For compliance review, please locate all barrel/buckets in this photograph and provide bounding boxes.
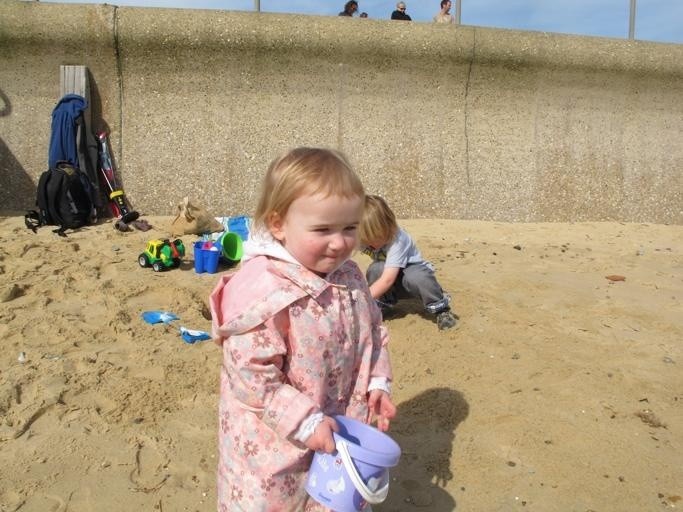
[214,232,243,267]
[301,414,403,512]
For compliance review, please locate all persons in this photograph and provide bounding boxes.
[359,192,459,332]
[338,1,368,19]
[433,0,456,23]
[390,2,411,20]
[208,147,399,512]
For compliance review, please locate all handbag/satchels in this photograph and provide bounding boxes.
[171,196,224,235]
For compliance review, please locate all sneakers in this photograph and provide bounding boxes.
[437,312,455,330]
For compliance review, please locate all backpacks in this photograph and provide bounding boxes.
[26,160,97,237]
